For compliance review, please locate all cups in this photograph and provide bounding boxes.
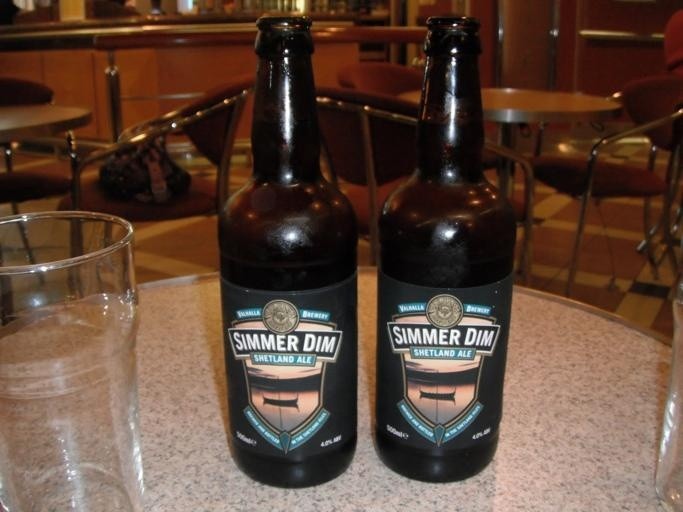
[655,299,683,511]
[1,210,148,511]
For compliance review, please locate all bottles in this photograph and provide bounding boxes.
[374,14,518,482]
[217,10,360,490]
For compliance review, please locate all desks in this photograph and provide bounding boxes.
[0,105,92,293]
[0,268,683,512]
[398,84,624,224]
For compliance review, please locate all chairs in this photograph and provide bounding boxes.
[535,76,682,298]
[339,61,518,196]
[1,79,53,283]
[314,86,545,280]
[55,75,258,292]
